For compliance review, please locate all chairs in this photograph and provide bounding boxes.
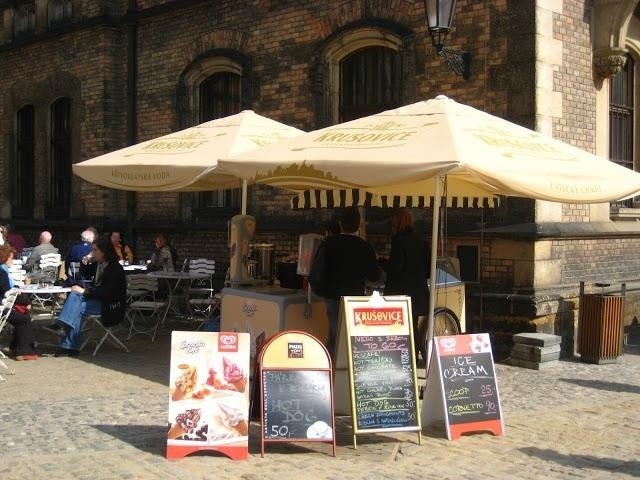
[1,247,230,381]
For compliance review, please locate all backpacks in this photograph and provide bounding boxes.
[308,235,336,298]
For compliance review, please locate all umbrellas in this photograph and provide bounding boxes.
[218,95,640,381]
[70,110,307,216]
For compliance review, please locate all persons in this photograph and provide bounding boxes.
[310,206,376,352]
[141,235,173,317]
[54,233,126,356]
[324,220,341,240]
[0,246,40,360]
[382,206,430,369]
[0,223,134,294]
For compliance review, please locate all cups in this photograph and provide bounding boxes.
[24,275,56,290]
[313,420,329,438]
[119,256,151,267]
[82,252,96,265]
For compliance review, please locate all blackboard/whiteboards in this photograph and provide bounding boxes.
[259,330,336,442]
[344,296,422,433]
[434,333,504,440]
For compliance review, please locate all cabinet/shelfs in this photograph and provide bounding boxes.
[365,254,467,343]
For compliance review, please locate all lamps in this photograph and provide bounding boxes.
[423,0,472,85]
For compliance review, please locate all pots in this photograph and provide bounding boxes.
[249,242,276,286]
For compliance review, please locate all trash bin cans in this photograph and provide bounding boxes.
[576,281,627,365]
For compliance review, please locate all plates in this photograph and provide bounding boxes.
[306,424,332,439]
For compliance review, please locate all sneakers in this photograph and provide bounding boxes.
[47,322,68,333]
[15,353,39,361]
[54,348,78,357]
